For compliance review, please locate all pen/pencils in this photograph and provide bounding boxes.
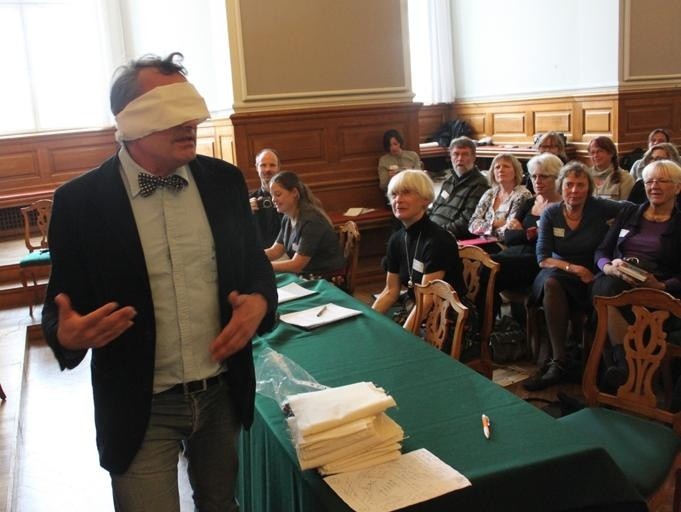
[317,307,326,317]
[481,414,491,440]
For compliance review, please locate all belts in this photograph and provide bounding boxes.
[160,375,221,395]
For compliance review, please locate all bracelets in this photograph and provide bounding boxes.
[493,220,497,228]
[566,262,572,273]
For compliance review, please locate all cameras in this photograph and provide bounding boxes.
[257,196,272,210]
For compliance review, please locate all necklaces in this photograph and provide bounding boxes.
[565,207,583,221]
[404,229,424,290]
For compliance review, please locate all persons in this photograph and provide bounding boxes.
[524,126,681,205]
[370,169,480,362]
[371,135,489,307]
[591,160,681,393]
[376,128,421,206]
[467,151,564,346]
[247,147,284,250]
[460,152,533,328]
[264,169,346,291]
[40,51,281,512]
[522,159,637,392]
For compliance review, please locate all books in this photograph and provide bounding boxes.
[274,282,319,304]
[278,302,364,331]
[617,261,649,282]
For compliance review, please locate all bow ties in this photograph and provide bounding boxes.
[137,172,189,198]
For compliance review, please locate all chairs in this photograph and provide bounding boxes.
[555,287,680,510]
[412,279,469,361]
[661,329,680,411]
[324,225,359,293]
[19,199,54,316]
[458,245,500,380]
[532,305,593,365]
[499,285,532,362]
[235,273,649,511]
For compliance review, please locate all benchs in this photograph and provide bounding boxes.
[327,206,393,235]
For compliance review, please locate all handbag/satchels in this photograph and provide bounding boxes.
[490,328,528,362]
[495,229,535,287]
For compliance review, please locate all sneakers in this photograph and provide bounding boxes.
[542,355,572,380]
[523,358,560,390]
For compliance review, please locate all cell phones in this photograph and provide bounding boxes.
[393,166,401,175]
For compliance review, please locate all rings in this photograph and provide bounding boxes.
[513,223,518,228]
